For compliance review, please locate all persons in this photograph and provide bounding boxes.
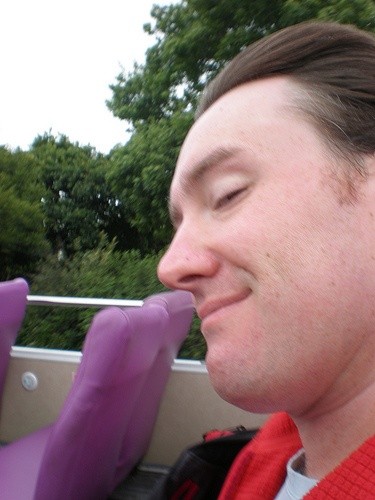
[148,21,375,500]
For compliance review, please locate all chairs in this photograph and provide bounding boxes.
[0,278,31,402]
[0,305,171,500]
[0,275,196,490]
